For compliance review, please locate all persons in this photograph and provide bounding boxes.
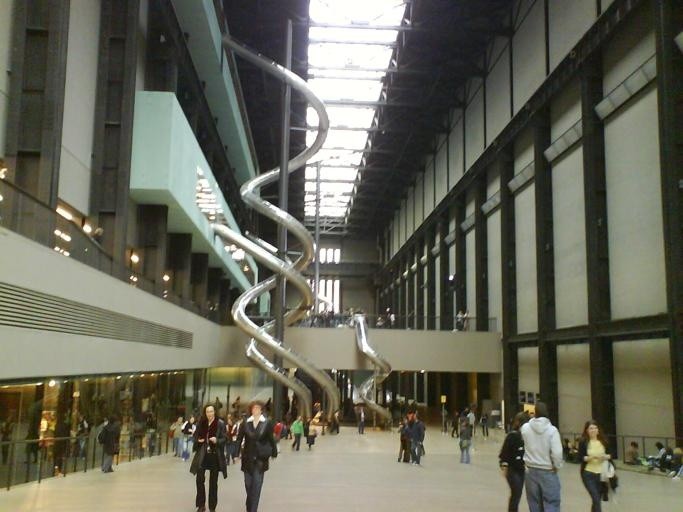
[448,402,491,465]
[191,405,228,512]
[307,306,471,332]
[499,400,564,511]
[2,411,93,475]
[560,436,584,463]
[100,415,122,473]
[171,402,323,464]
[328,408,342,434]
[393,406,430,463]
[625,438,683,481]
[233,402,279,512]
[125,409,165,461]
[356,403,369,436]
[577,417,618,512]
